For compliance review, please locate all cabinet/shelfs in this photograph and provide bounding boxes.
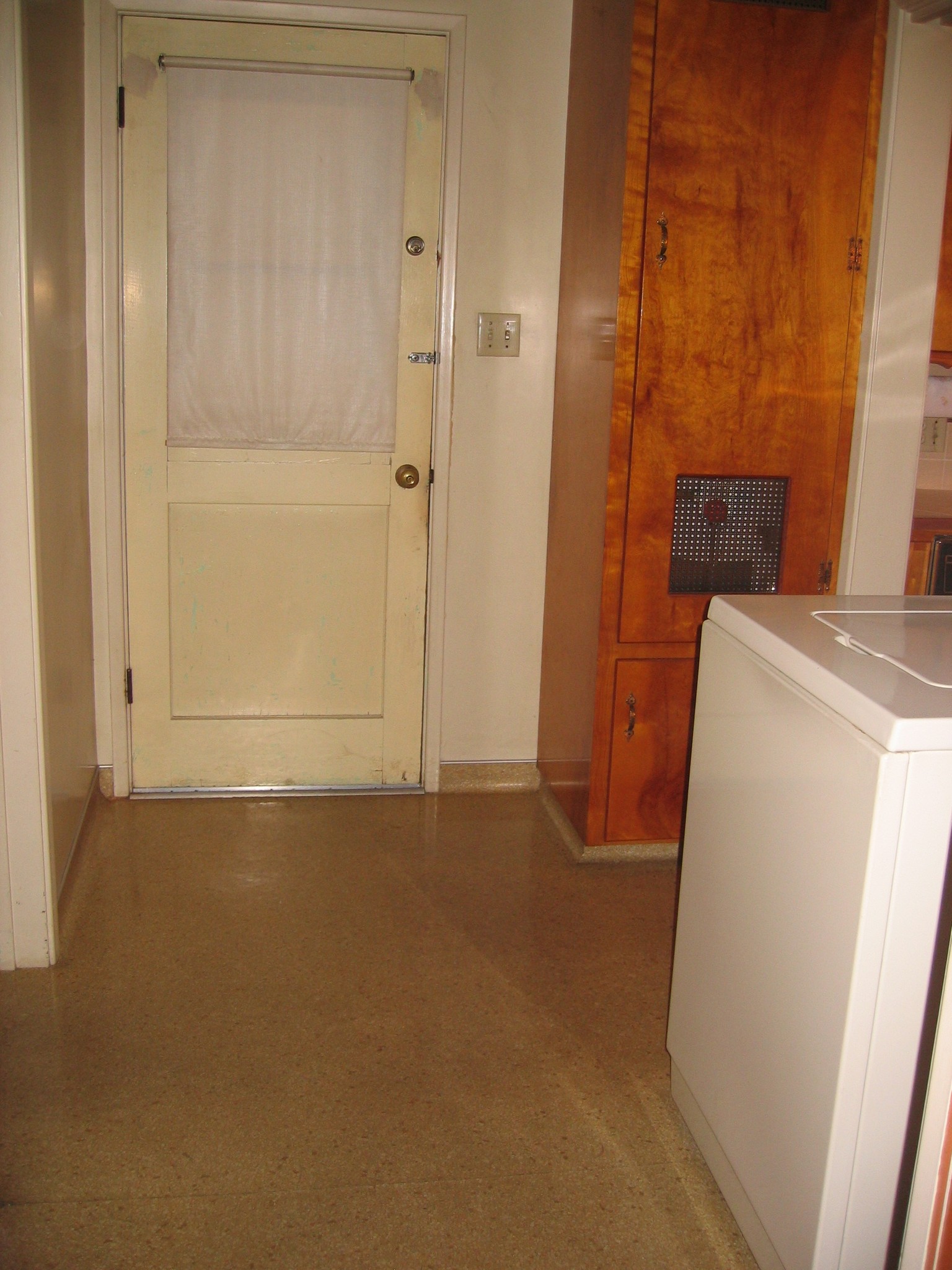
[535,0,885,848]
